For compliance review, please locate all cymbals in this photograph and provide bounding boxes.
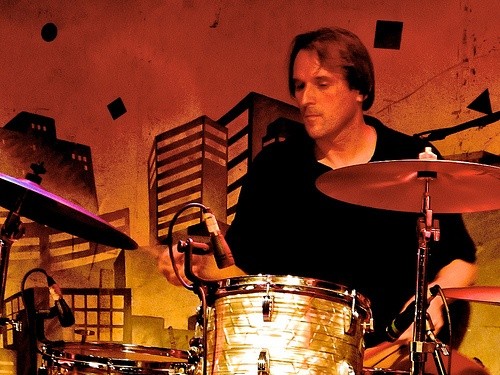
[0,171,138,251]
[314,158,500,214]
[438,286,500,307]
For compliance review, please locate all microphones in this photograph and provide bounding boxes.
[202,207,235,269]
[47,276,76,327]
[385,285,440,343]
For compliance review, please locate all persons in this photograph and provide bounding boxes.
[160,26,480,375]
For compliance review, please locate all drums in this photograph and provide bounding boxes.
[36,340,200,375]
[362,366,413,375]
[182,274,375,375]
